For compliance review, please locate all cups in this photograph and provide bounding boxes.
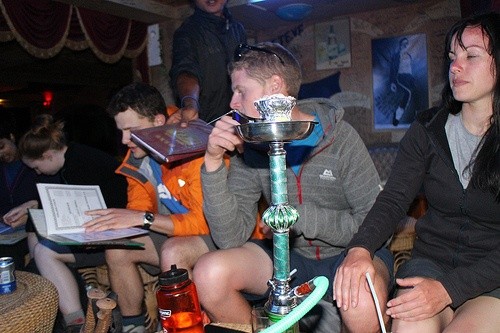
[251,307,270,333]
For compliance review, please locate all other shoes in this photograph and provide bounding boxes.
[113,324,146,333]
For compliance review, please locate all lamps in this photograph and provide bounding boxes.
[276,3,313,20]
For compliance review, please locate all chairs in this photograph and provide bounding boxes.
[80,145,418,333]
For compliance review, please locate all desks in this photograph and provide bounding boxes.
[0,269,59,333]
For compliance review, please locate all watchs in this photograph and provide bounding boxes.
[143,210,154,229]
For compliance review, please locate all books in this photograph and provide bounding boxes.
[130,117,214,164]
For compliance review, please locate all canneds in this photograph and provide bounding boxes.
[0,257,16,295]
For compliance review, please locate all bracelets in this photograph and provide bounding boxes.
[180,96,197,108]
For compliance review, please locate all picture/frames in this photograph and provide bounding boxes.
[313,17,353,73]
[368,30,432,133]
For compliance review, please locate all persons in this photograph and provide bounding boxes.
[82,82,275,333]
[186,42,396,333]
[0,115,128,333]
[165,0,248,130]
[332,7,500,333]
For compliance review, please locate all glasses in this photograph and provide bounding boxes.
[233,42,286,66]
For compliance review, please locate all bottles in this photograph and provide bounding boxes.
[157,265,206,333]
